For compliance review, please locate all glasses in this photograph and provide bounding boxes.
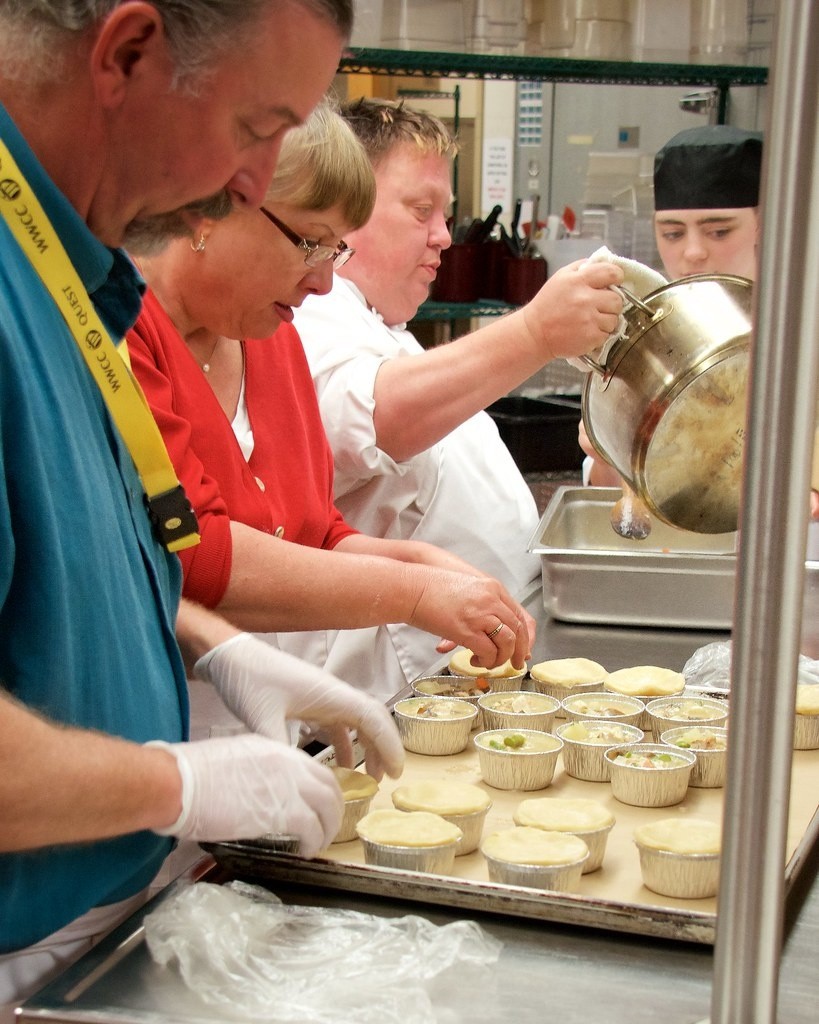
[261,205,355,270]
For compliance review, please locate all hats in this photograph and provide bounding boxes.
[652,124,764,210]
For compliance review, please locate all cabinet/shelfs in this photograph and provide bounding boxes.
[327,48,770,319]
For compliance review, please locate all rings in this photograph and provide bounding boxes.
[488,622,504,638]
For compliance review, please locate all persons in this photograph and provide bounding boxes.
[290,94,626,686]
[576,124,819,520]
[0,0,407,1018]
[126,94,536,733]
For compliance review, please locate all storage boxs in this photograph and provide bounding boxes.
[484,390,586,469]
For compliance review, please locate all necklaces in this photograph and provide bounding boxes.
[136,252,222,372]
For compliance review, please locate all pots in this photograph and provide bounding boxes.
[577,273,753,535]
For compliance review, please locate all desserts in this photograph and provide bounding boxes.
[396,648,818,808]
[326,764,722,897]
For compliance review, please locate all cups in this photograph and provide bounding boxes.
[428,245,483,302]
[503,257,548,304]
[481,240,520,297]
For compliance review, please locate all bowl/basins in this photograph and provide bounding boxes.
[446,665,528,692]
[359,793,493,876]
[556,721,645,782]
[635,839,721,898]
[791,713,819,749]
[529,669,603,717]
[474,729,563,790]
[478,693,561,731]
[562,692,645,730]
[480,816,616,893]
[331,787,378,844]
[411,675,493,729]
[606,685,731,808]
[393,696,478,755]
